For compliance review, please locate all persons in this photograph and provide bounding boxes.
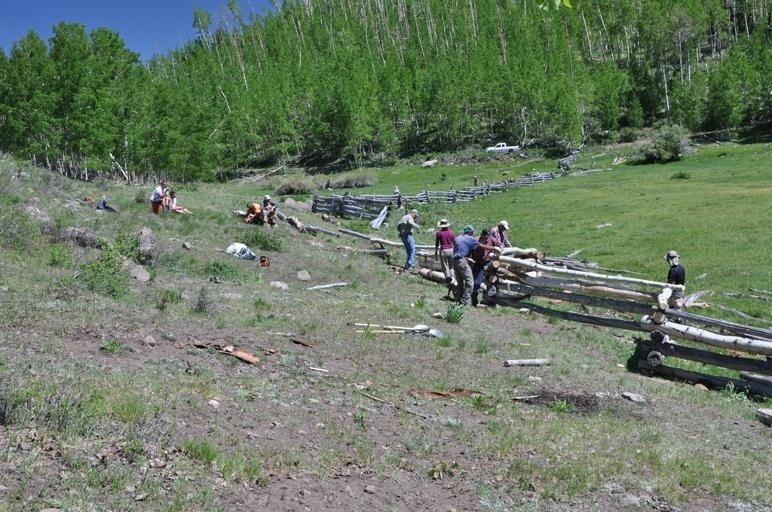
[397,206,513,311]
[149,180,170,214]
[262,194,277,227]
[245,203,261,223]
[168,191,194,214]
[662,250,685,323]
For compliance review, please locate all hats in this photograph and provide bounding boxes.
[264,194,271,201]
[411,209,419,217]
[664,250,681,261]
[437,219,451,228]
[499,220,510,230]
[480,228,491,235]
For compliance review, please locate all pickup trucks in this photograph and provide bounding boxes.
[486,143,519,153]
